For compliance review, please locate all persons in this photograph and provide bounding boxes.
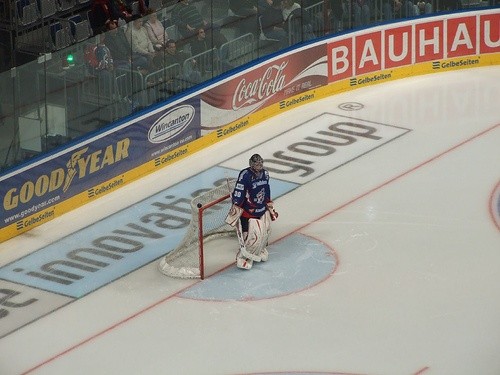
[86,0,463,109]
[230,153,279,271]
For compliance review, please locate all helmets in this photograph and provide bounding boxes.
[250,154,264,177]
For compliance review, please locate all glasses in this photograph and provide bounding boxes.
[199,31,205,34]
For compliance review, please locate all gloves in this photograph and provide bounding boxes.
[267,203,278,221]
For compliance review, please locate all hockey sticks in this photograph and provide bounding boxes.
[227,179,263,263]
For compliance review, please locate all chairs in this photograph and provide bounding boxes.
[17,0,281,105]
